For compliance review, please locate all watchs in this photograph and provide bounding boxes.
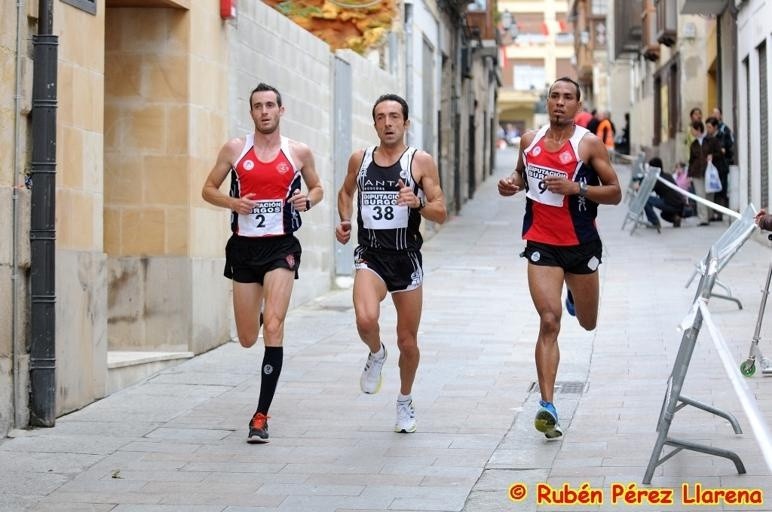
[579,179,588,197]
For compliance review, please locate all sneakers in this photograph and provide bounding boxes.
[360,342,386,394]
[647,224,660,228]
[394,400,416,433]
[566,289,575,315]
[697,217,722,225]
[535,401,562,438]
[247,413,269,443]
[673,215,680,226]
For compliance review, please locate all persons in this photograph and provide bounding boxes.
[635,105,734,228]
[575,106,631,155]
[496,75,624,441]
[202,81,324,446]
[335,94,448,433]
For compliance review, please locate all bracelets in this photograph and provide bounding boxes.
[414,197,426,211]
[304,198,312,212]
